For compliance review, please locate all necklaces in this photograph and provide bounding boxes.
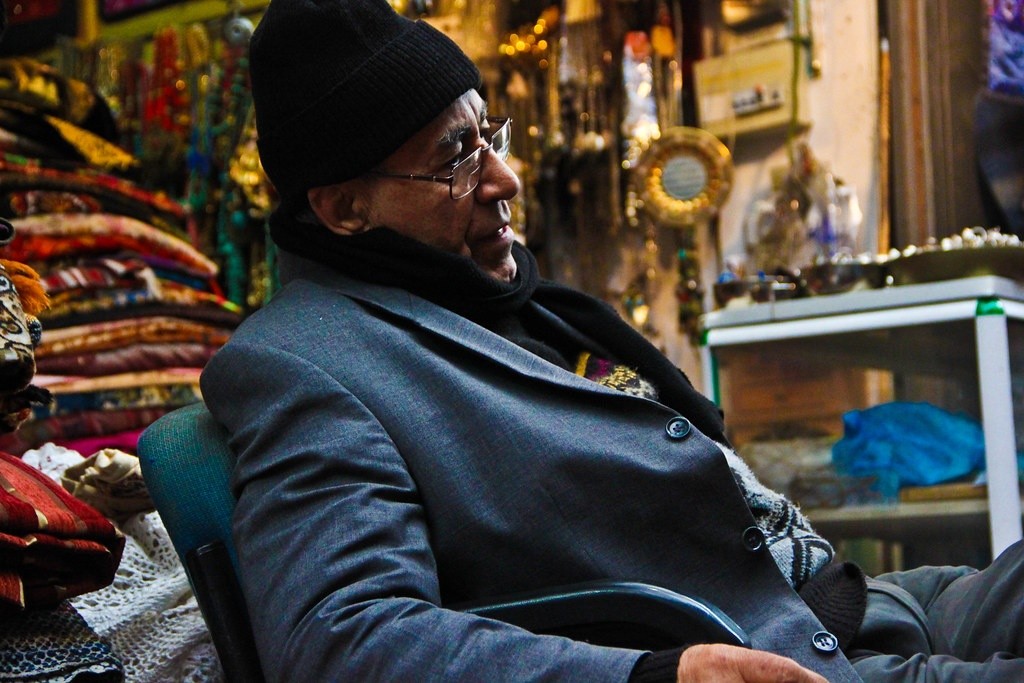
[51,1,714,347]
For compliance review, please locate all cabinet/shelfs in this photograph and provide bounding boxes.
[699,275,1024,562]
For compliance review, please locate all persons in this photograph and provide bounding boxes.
[198,1,1024,683]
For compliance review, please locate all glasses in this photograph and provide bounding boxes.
[371,115,512,200]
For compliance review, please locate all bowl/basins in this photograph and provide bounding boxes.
[712,243,1024,308]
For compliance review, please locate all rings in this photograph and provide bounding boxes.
[817,222,1022,266]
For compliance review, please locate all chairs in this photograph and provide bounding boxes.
[139,403,753,683]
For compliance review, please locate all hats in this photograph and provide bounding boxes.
[249,0,481,194]
